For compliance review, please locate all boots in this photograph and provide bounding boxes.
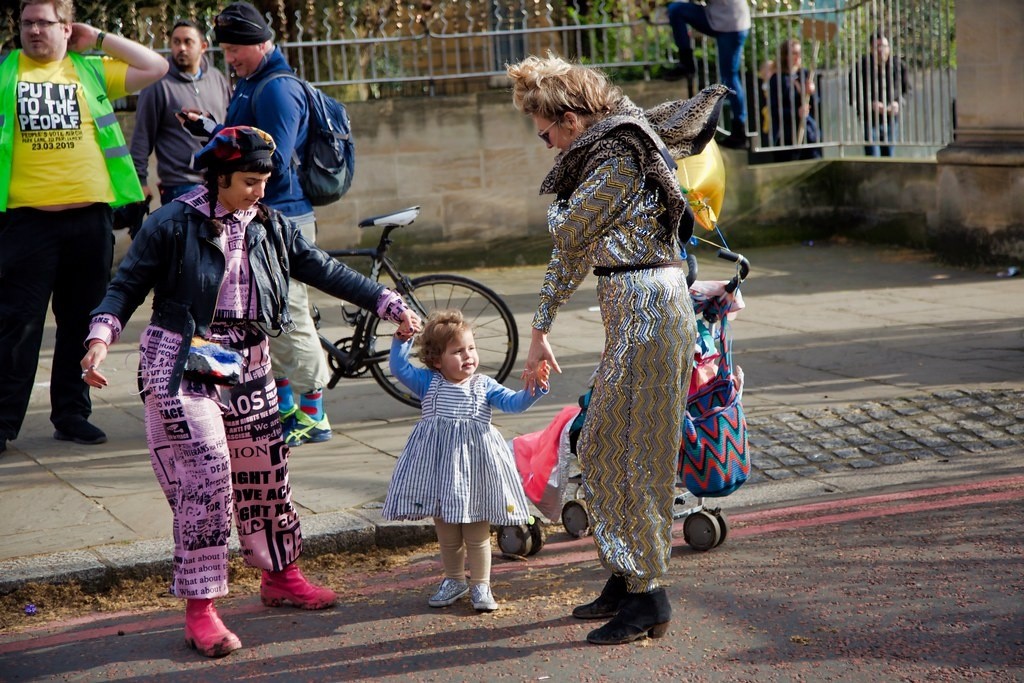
[680,49,695,79]
[719,119,750,149]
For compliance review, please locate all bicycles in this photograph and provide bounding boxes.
[136,203,520,410]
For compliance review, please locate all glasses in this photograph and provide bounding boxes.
[17,17,64,30]
[212,14,262,30]
[538,109,576,144]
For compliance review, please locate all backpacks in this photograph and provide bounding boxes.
[683,320,722,396]
[252,69,354,206]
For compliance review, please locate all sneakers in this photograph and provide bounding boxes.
[429,577,469,606]
[278,403,299,432]
[281,410,333,447]
[54,418,107,444]
[470,584,498,610]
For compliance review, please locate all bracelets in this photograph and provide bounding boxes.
[96,31,107,51]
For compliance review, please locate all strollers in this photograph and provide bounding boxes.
[488,247,754,561]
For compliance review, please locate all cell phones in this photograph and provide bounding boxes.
[178,112,190,120]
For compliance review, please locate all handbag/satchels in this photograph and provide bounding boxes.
[676,380,751,498]
[184,337,244,387]
[805,113,822,142]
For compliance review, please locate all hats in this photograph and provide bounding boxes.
[192,125,276,174]
[215,2,272,45]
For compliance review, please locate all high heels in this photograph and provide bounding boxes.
[260,561,337,609]
[573,573,634,620]
[587,588,671,644]
[184,598,243,658]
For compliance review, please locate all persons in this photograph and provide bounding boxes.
[747,38,820,167]
[129,20,234,207]
[848,28,913,157]
[79,125,420,657]
[662,0,751,148]
[0,0,169,452]
[382,310,551,610]
[175,2,331,446]
[507,55,735,642]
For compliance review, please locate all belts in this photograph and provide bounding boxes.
[593,261,682,276]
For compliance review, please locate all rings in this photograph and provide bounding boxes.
[84,369,89,373]
[81,373,86,379]
[524,369,528,372]
